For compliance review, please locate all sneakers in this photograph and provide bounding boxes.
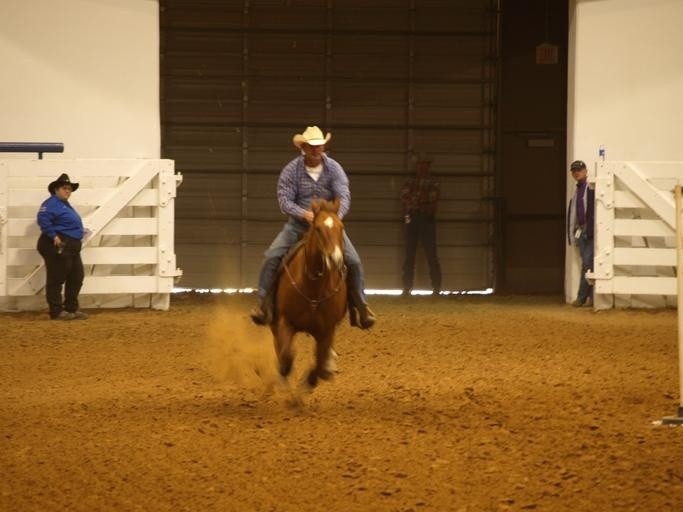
[252,296,267,323]
[55,311,90,320]
[350,308,376,326]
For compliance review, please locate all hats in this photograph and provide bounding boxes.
[293,126,331,149]
[48,174,79,195]
[571,161,586,170]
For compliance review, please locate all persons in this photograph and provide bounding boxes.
[249,126,376,327]
[35,173,89,320]
[566,159,594,307]
[397,150,442,297]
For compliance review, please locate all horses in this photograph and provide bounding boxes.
[268,196,349,391]
